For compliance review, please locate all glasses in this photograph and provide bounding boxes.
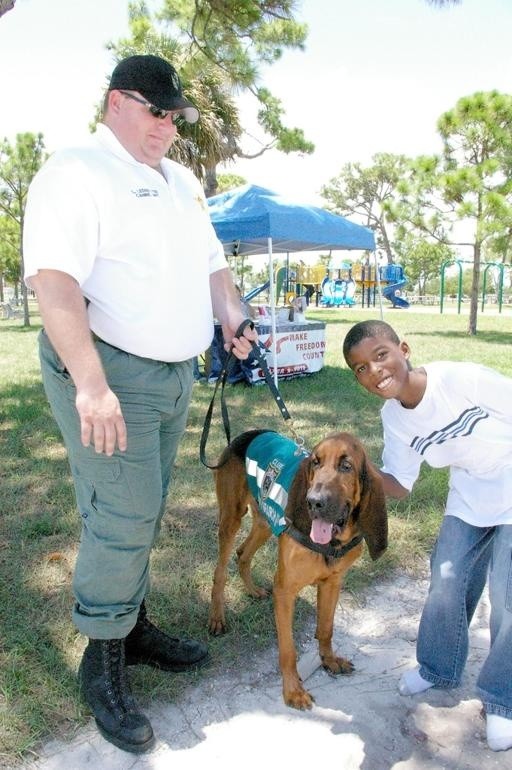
[119,90,186,127]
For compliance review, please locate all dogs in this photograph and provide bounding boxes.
[206,428,388,712]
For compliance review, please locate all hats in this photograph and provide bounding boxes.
[108,54,201,124]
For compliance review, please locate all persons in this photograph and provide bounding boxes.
[22,56,258,753]
[343,320,512,750]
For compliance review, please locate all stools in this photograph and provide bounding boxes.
[195,351,213,382]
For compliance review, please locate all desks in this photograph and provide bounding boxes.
[207,318,326,387]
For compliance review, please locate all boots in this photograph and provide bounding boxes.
[77,637,155,754]
[123,597,211,674]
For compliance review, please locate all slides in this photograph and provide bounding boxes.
[381,277,410,309]
[320,276,357,305]
